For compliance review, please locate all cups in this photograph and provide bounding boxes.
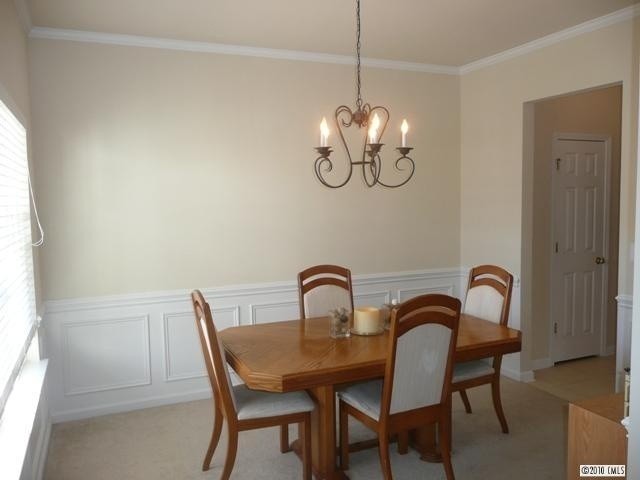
[354,307,379,332]
[329,308,351,339]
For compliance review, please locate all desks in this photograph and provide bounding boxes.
[566,393,629,480]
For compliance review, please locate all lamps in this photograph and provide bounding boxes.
[314,0,415,188]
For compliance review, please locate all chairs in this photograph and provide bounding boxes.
[449,264,513,451]
[190,290,317,480]
[298,264,353,319]
[337,293,462,480]
